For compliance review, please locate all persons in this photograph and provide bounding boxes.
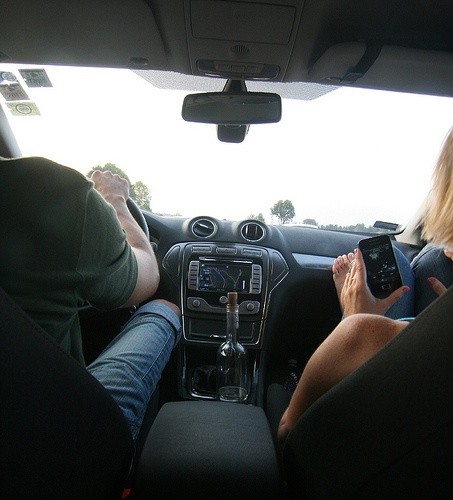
[0,156,182,443]
[277,126,453,445]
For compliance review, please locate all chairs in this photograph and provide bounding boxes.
[283,286,453,500]
[0,287,135,500]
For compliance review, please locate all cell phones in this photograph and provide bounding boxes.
[358,235,403,299]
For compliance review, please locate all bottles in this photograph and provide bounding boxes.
[219,293,249,402]
